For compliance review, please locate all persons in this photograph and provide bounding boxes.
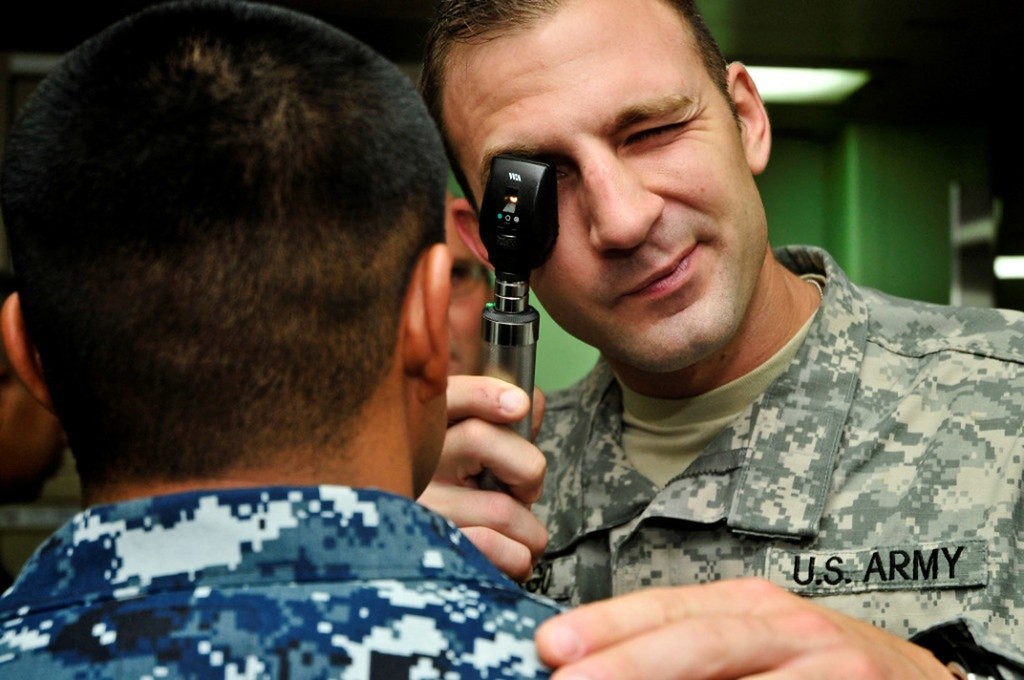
[445,187,497,378]
[417,0,1024,680]
[2,266,72,508]
[0,0,561,680]
